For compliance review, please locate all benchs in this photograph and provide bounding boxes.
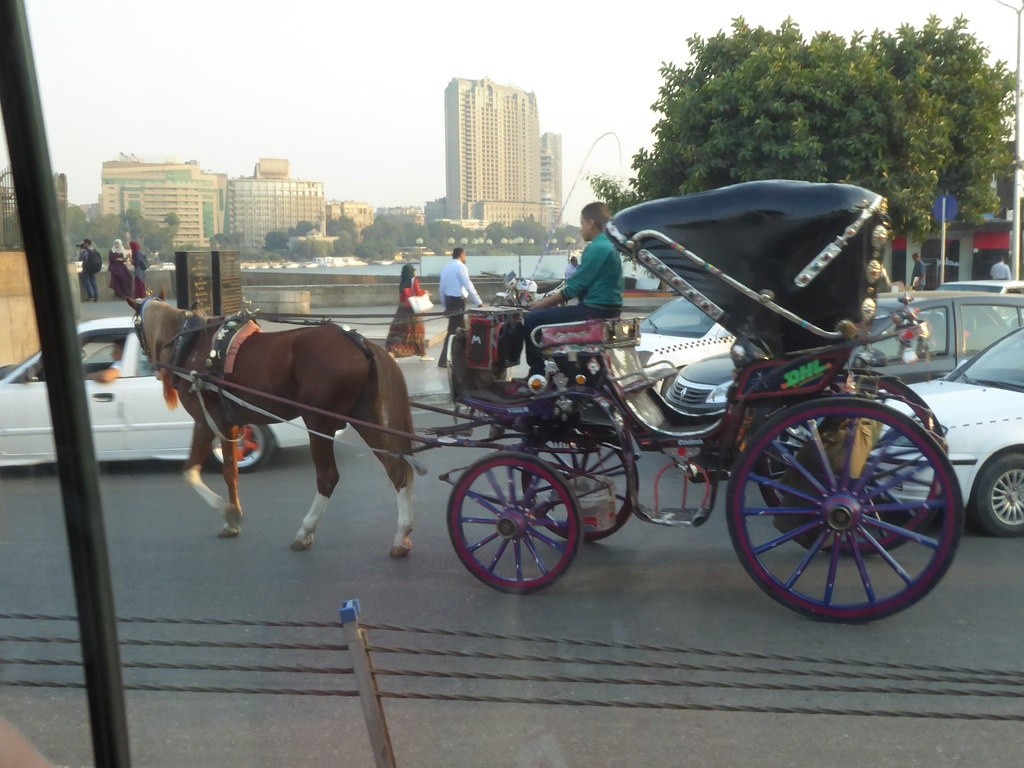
[532,315,643,350]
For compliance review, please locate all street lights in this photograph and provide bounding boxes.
[448,236,576,278]
[415,237,423,275]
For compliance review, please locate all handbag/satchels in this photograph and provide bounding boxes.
[408,278,435,314]
[140,256,150,270]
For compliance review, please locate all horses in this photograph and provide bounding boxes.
[127,289,414,557]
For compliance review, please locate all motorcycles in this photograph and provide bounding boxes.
[490,271,568,311]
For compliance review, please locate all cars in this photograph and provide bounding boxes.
[798,323,1024,536]
[665,289,1024,415]
[636,292,737,373]
[0,317,349,473]
[935,280,1024,323]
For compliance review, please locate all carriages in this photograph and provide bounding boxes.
[123,183,966,624]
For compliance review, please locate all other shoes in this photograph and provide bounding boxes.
[87,297,97,302]
[421,356,434,360]
[513,371,544,385]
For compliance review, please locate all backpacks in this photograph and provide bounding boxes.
[85,248,102,272]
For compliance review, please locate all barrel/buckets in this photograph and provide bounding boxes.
[550,476,616,534]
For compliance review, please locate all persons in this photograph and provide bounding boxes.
[437,247,483,368]
[524,202,623,376]
[108,239,133,298]
[86,337,126,381]
[565,256,579,279]
[990,257,1012,280]
[79,238,99,302]
[385,264,435,361]
[910,254,926,290]
[128,240,146,297]
